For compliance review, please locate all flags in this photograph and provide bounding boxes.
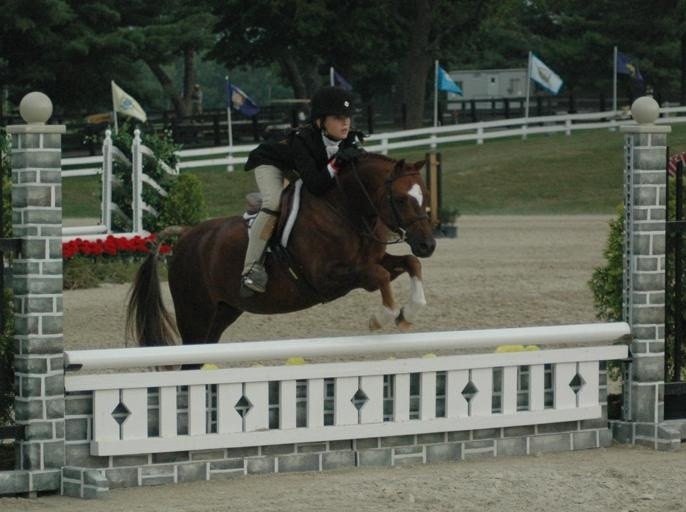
[226,80,260,117]
[111,81,147,124]
[531,54,564,95]
[438,65,464,97]
[334,71,353,91]
[617,51,644,81]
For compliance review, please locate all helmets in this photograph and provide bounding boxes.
[309,86,353,117]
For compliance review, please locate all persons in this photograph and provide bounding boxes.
[238,86,358,294]
[191,84,204,123]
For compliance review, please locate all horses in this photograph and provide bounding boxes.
[124,152,436,390]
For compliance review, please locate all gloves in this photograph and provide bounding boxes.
[328,148,364,178]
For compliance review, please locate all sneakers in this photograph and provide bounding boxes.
[241,259,270,294]
